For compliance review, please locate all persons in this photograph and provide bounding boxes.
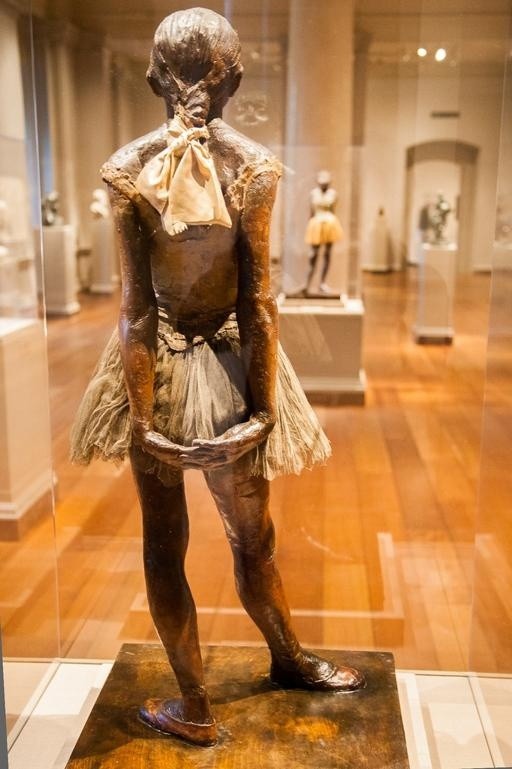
[44,190,64,224]
[299,166,344,302]
[62,8,372,743]
[89,186,107,217]
